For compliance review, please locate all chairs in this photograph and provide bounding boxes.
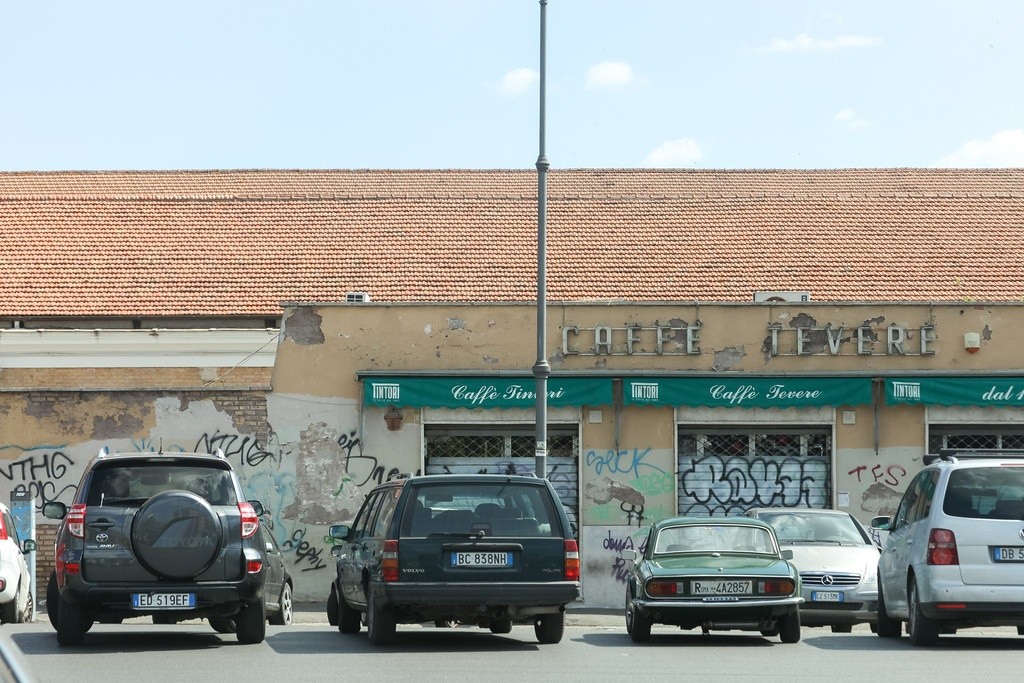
[411,500,541,535]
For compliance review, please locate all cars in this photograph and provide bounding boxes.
[0,502,36,624]
[152,521,294,634]
[743,509,883,638]
[627,516,806,643]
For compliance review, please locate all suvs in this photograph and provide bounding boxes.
[328,474,582,645]
[872,449,1023,647]
[44,445,266,646]
